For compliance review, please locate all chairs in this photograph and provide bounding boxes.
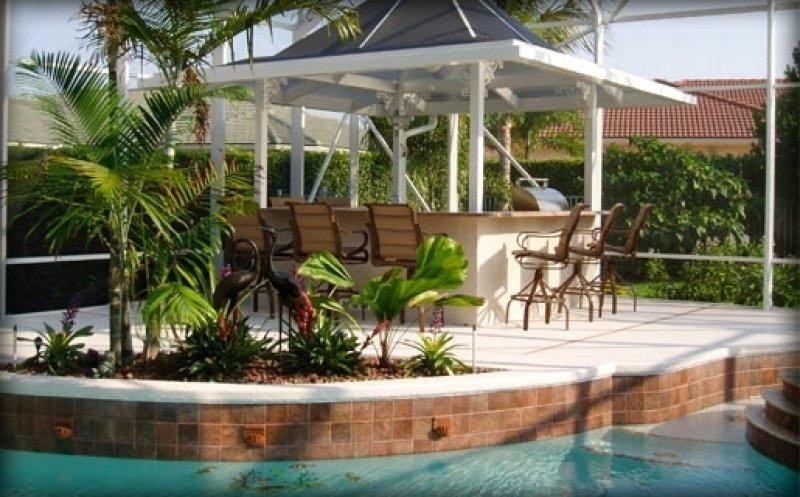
[504,202,659,332]
[214,195,450,334]
